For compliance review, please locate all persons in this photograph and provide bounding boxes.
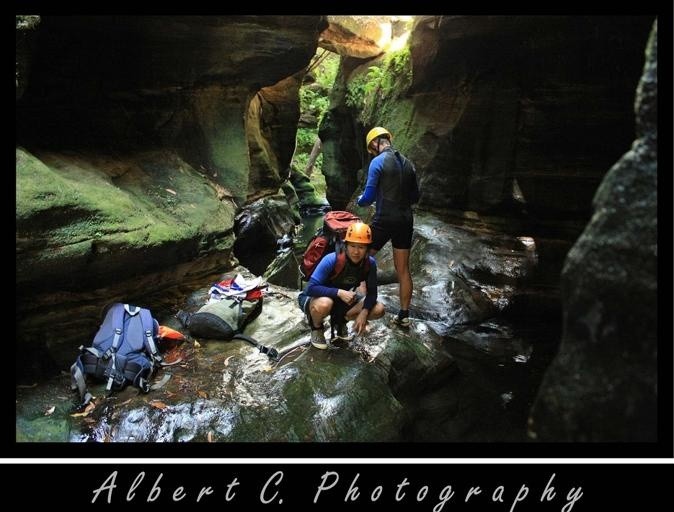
[295,222,385,351]
[357,127,421,328]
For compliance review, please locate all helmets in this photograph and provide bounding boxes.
[345,223,373,244]
[366,127,391,154]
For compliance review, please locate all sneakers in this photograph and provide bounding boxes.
[393,309,410,326]
[311,328,327,349]
[335,322,354,340]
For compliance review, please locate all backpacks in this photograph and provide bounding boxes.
[297,211,361,291]
[82,303,159,385]
[187,273,263,342]
